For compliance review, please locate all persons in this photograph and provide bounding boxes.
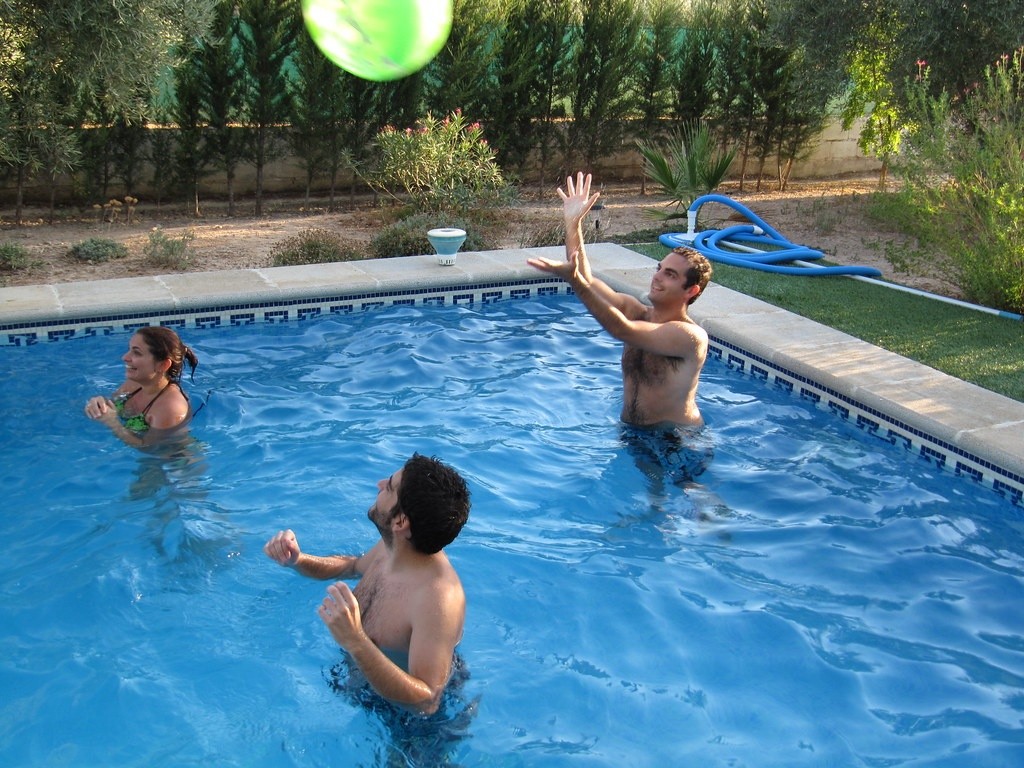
[263,450,474,716]
[83,326,199,448]
[526,170,713,430]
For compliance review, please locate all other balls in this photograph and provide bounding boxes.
[299,0,454,82]
